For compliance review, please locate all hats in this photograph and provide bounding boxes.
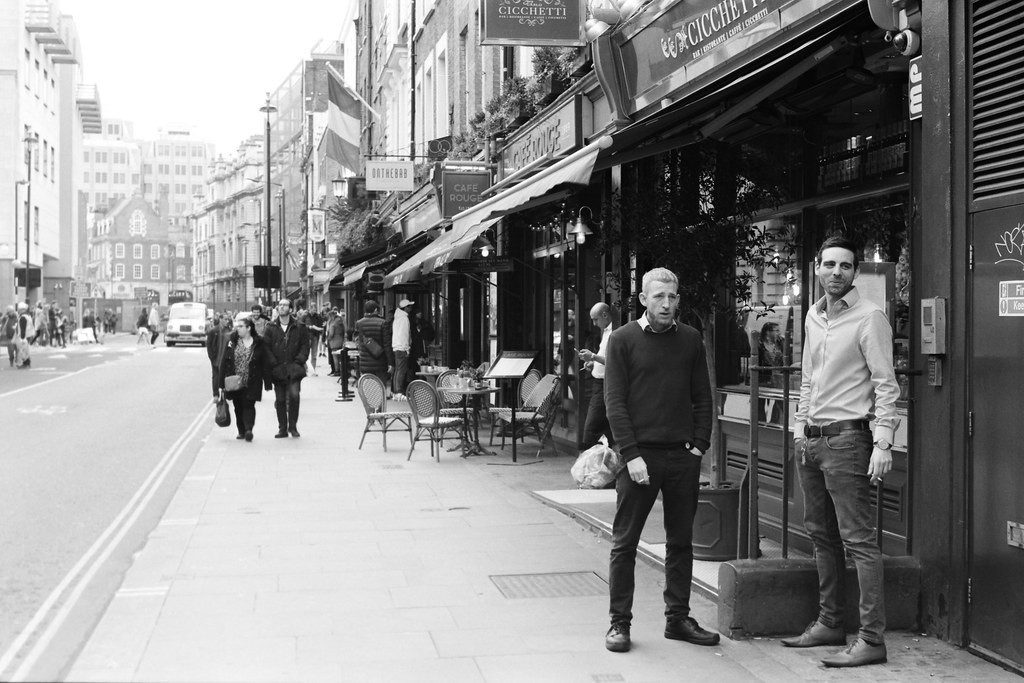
[398,300,415,309]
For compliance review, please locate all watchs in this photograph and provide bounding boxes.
[873,438,893,450]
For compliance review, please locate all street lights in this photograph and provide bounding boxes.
[246,178,287,299]
[24,137,39,304]
[258,106,279,317]
[274,194,283,300]
[16,179,29,260]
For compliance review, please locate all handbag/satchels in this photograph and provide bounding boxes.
[214,393,231,428]
[223,374,244,393]
[363,335,386,361]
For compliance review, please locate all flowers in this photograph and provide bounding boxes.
[418,356,430,366]
[469,368,482,382]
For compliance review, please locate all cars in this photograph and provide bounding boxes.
[164,302,213,347]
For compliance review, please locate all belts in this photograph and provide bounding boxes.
[802,420,871,439]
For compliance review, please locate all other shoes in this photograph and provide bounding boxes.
[314,372,319,377]
[275,430,288,438]
[318,353,327,358]
[236,429,254,441]
[286,426,300,438]
[387,392,410,402]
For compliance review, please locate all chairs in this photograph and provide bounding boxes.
[358,365,562,461]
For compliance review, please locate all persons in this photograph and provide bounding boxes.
[136,302,160,349]
[206,297,310,441]
[82,308,118,345]
[757,321,784,424]
[780,237,901,668]
[0,301,37,368]
[386,299,435,401]
[28,299,70,348]
[355,300,395,390]
[604,266,720,653]
[579,302,613,453]
[294,302,346,377]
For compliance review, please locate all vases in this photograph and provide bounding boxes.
[420,364,427,371]
[474,380,483,391]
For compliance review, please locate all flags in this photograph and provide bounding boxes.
[327,72,361,175]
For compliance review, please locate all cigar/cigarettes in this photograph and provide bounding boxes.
[639,476,650,483]
[580,368,585,371]
[872,474,882,481]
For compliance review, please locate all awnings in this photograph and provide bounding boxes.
[342,232,429,287]
[452,24,845,244]
[323,272,344,294]
[383,213,578,296]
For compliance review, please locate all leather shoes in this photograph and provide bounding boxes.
[781,621,848,648]
[665,615,721,646]
[820,638,888,666]
[606,620,632,653]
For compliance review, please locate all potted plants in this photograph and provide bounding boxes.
[457,37,592,159]
[632,140,790,562]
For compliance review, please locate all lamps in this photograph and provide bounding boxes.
[475,227,498,259]
[569,205,605,245]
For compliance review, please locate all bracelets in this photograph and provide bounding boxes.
[590,353,595,361]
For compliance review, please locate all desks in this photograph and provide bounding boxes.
[415,371,444,387]
[435,386,501,457]
